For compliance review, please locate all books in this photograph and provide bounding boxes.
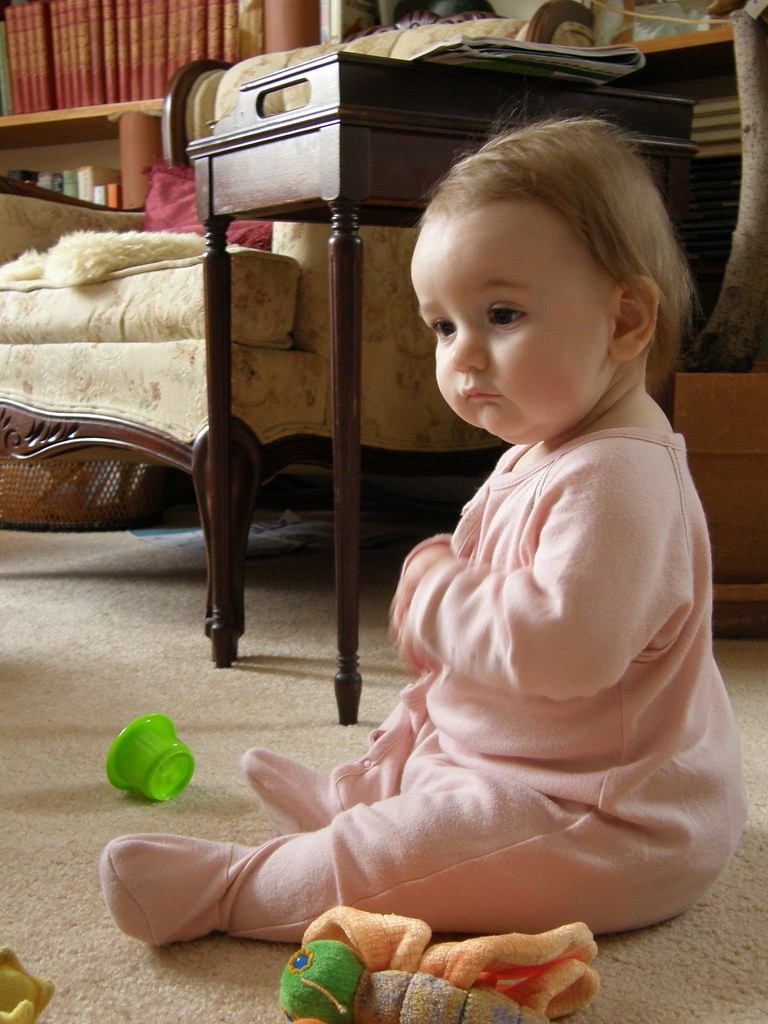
[320,0,378,44]
[0,0,265,115]
[8,167,122,208]
[410,33,645,85]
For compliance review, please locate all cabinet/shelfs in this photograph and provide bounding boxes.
[0,0,328,209]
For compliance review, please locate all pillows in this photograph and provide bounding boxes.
[140,159,272,250]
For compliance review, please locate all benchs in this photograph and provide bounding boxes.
[0,0,596,636]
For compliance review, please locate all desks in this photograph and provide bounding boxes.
[187,50,702,725]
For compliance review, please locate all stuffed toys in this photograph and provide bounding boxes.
[0,947,55,1024]
[281,906,601,1024]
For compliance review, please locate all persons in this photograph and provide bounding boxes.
[102,121,743,945]
